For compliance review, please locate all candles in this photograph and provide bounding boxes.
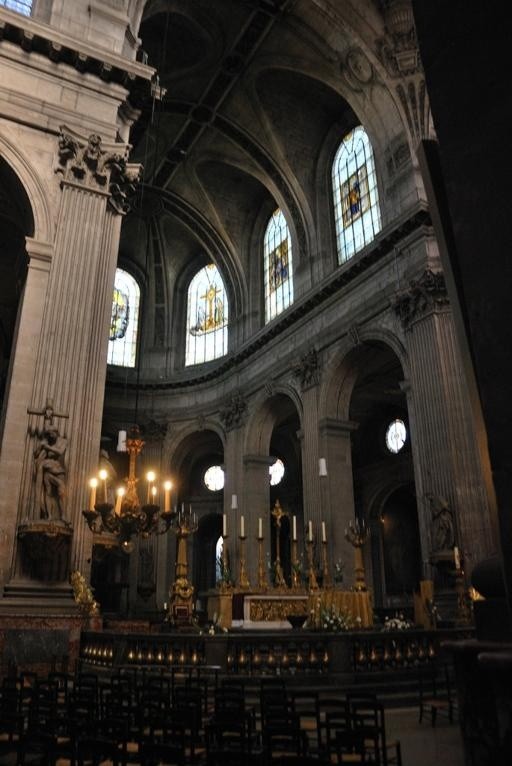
[220,512,263,539]
[292,515,327,542]
[90,470,171,517]
